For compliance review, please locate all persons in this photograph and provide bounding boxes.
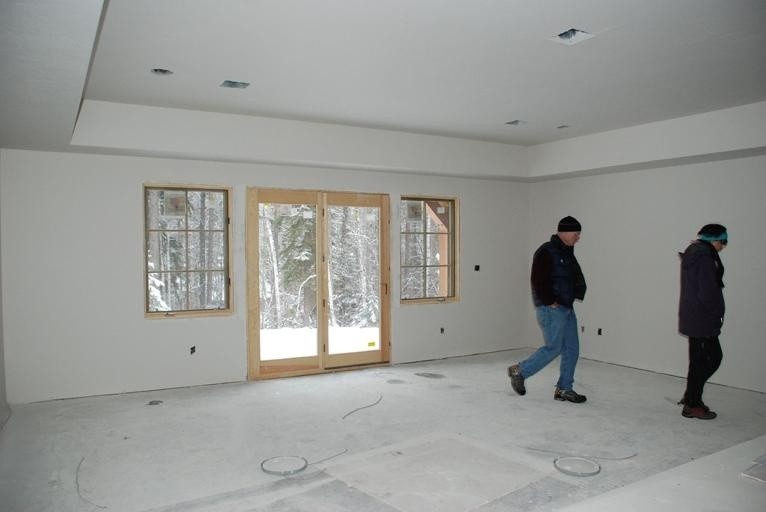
[679,223,728,420]
[507,216,587,403]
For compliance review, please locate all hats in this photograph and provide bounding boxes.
[558,217,581,232]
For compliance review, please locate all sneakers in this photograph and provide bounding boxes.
[681,399,717,419]
[509,364,526,395]
[554,387,586,403]
